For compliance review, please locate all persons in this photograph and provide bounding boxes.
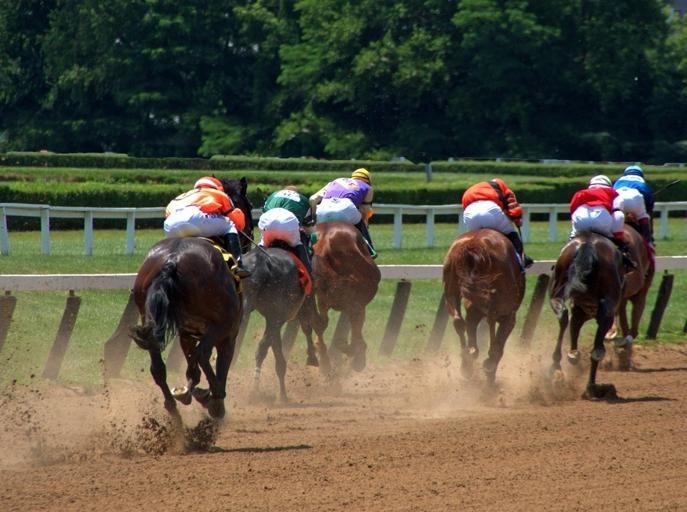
[163,176,251,279]
[461,178,533,270]
[569,175,637,269]
[613,165,655,254]
[258,186,313,277]
[308,168,378,259]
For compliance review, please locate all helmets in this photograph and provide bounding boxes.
[194,177,224,191]
[624,166,644,177]
[590,175,613,186]
[352,168,372,186]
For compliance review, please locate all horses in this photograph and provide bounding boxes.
[547,231,625,400]
[310,220,380,375]
[441,226,525,385]
[604,218,656,348]
[235,213,319,404]
[127,172,254,428]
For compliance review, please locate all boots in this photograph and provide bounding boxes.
[637,215,656,251]
[294,244,312,275]
[224,233,251,278]
[355,219,377,259]
[507,232,533,269]
